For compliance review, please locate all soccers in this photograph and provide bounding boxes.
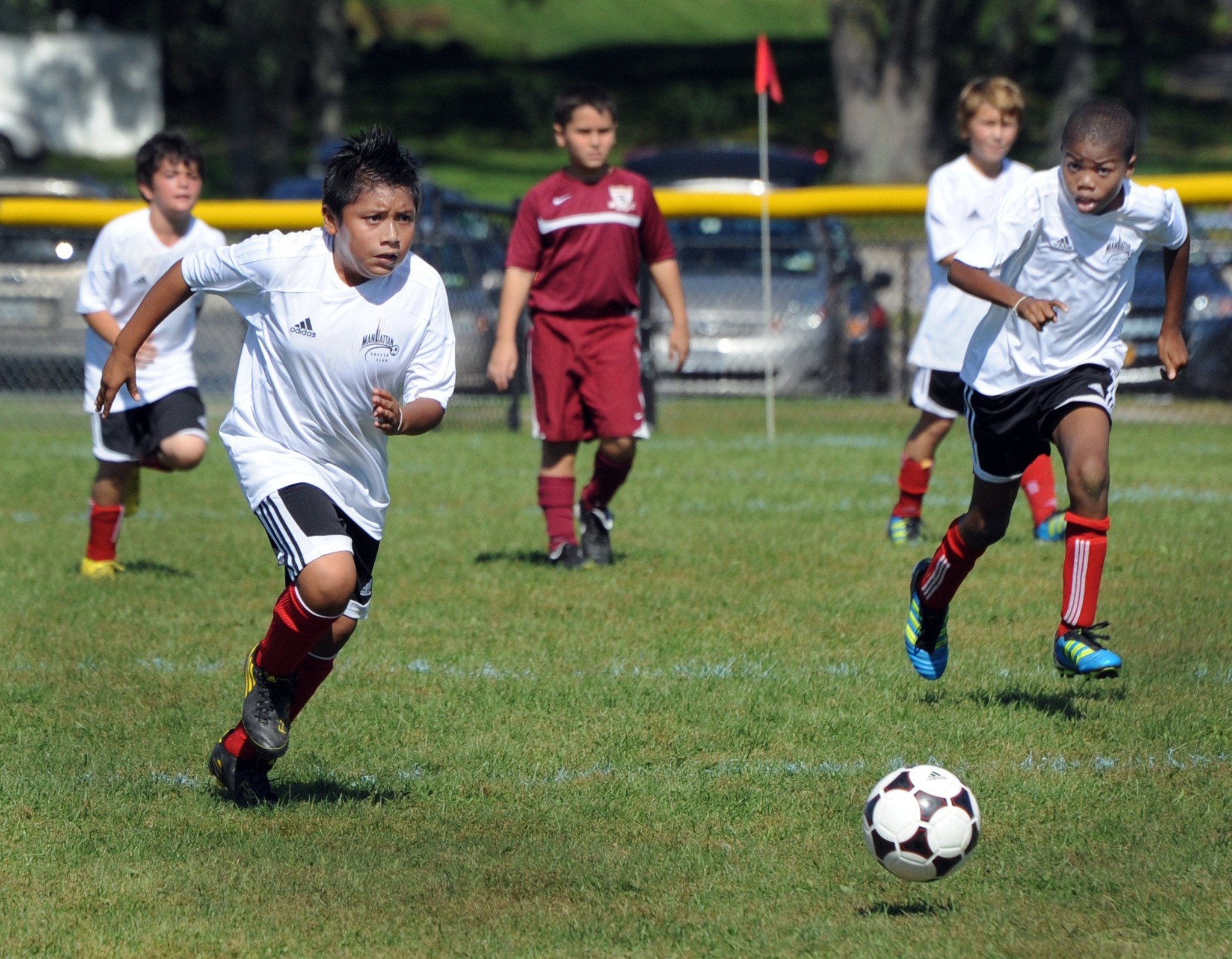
[861,764,982,883]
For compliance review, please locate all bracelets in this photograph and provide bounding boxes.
[1013,295,1033,319]
[395,406,403,433]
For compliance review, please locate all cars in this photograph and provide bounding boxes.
[269,144,896,402]
[1116,207,1232,398]
[3,177,122,362]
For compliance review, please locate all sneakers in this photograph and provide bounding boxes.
[1052,620,1123,679]
[242,643,290,758]
[208,728,274,806]
[573,499,614,564]
[890,513,928,549]
[905,559,950,680]
[548,540,582,570]
[1032,509,1068,543]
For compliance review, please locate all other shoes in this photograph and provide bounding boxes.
[79,556,125,583]
[122,464,141,516]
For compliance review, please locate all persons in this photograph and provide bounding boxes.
[74,135,228,585]
[95,132,454,811]
[888,76,1076,550]
[488,86,691,571]
[902,96,1188,678]
